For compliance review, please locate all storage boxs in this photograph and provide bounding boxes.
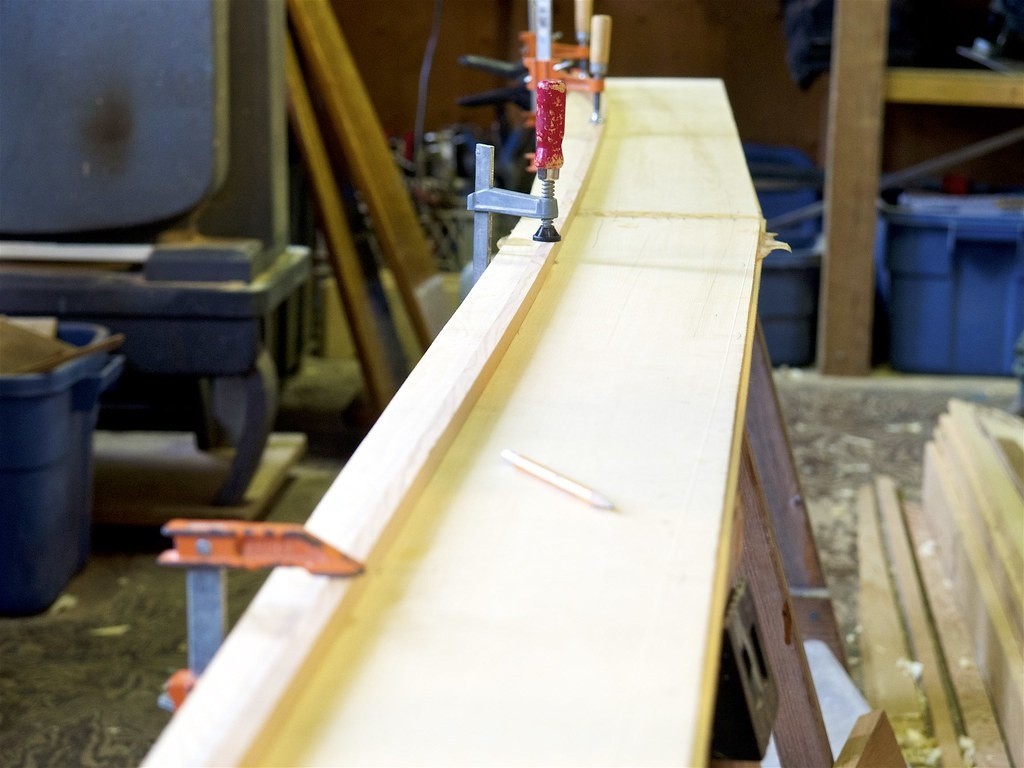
[0,323,126,618]
[874,210,1024,376]
